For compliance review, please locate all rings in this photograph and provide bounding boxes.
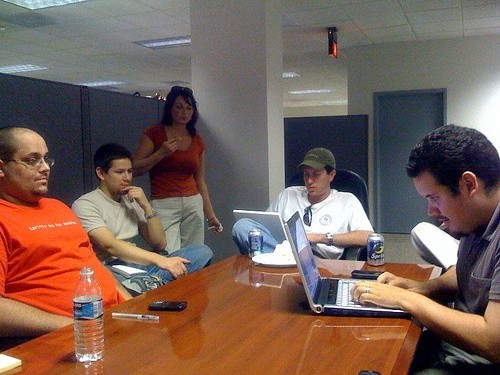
[365,287,370,293]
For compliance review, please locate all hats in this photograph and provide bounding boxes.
[298,148,336,169]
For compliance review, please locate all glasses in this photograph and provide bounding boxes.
[2,156,54,169]
[172,86,193,95]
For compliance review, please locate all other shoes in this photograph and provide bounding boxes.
[121,275,164,294]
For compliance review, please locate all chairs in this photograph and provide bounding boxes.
[287,169,369,224]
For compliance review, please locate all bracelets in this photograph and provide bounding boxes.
[143,208,157,222]
[326,232,334,246]
[207,215,215,222]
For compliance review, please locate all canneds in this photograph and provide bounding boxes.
[367,233,385,266]
[248,230,263,257]
[248,260,264,287]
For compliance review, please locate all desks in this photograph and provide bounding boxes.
[0,257,442,375]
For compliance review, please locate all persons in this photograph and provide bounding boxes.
[411,220,462,272]
[231,148,375,260]
[349,124,500,375]
[71,144,214,299]
[0,126,134,337]
[131,86,224,254]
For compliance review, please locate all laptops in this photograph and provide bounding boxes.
[284,211,413,319]
[233,210,287,244]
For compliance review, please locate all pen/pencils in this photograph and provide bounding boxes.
[112,313,159,320]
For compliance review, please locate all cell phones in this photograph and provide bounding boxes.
[148,300,188,312]
[351,269,384,280]
